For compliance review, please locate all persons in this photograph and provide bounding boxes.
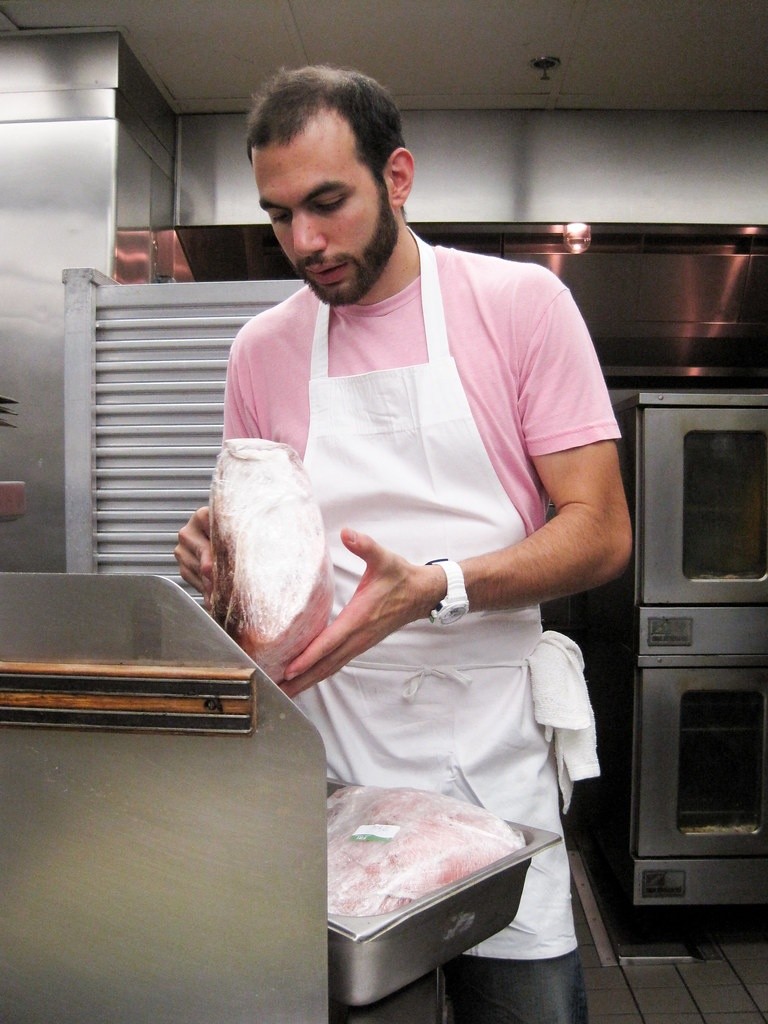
[174,66,634,1024]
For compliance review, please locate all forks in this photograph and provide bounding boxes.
[0,395,21,429]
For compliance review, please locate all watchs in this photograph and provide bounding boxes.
[424,558,469,626]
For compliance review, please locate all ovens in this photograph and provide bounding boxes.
[586,393,768,906]
